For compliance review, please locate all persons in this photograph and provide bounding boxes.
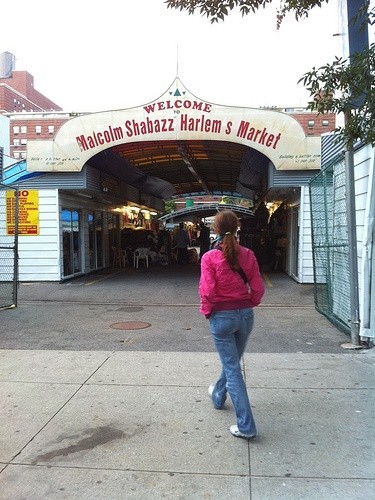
[198,210,265,438]
[273,234,289,272]
[175,221,210,265]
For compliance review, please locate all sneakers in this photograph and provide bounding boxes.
[230,425,255,438]
[208,385,214,397]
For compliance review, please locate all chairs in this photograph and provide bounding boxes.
[112,248,149,269]
